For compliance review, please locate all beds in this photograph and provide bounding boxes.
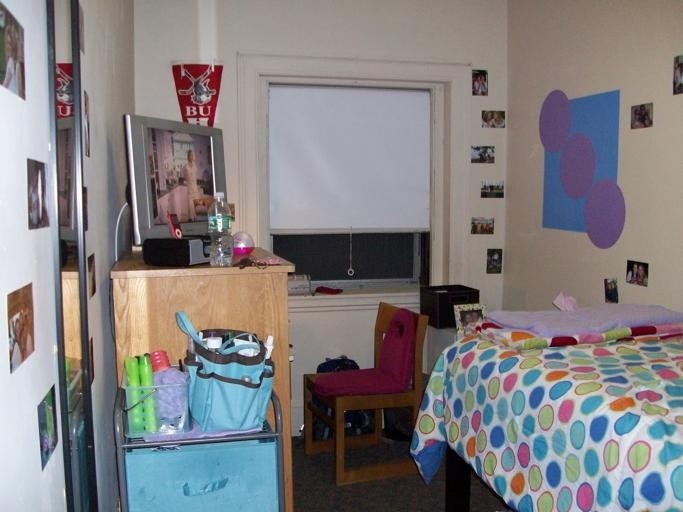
[445,332,681,511]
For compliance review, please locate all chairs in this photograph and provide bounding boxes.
[303,302,430,486]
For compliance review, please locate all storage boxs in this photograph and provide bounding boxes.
[419,284,479,329]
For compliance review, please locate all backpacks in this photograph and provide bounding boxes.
[315,355,372,440]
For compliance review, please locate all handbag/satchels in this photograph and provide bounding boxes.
[183,329,274,432]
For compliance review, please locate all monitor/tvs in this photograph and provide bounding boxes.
[123,113,228,247]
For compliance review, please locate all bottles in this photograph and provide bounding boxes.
[206,192,233,268]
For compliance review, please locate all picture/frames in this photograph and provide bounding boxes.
[455,304,487,330]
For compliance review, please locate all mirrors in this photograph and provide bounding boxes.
[45,0,96,512]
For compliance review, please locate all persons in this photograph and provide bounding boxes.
[632,108,646,129]
[0,22,27,96]
[178,148,203,223]
[490,249,498,272]
[462,310,473,326]
[674,63,683,95]
[638,105,650,128]
[472,73,505,165]
[470,218,495,234]
[606,262,648,303]
[9,303,35,372]
[27,164,45,228]
[467,311,480,327]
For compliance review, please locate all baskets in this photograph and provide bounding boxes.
[121,365,189,438]
